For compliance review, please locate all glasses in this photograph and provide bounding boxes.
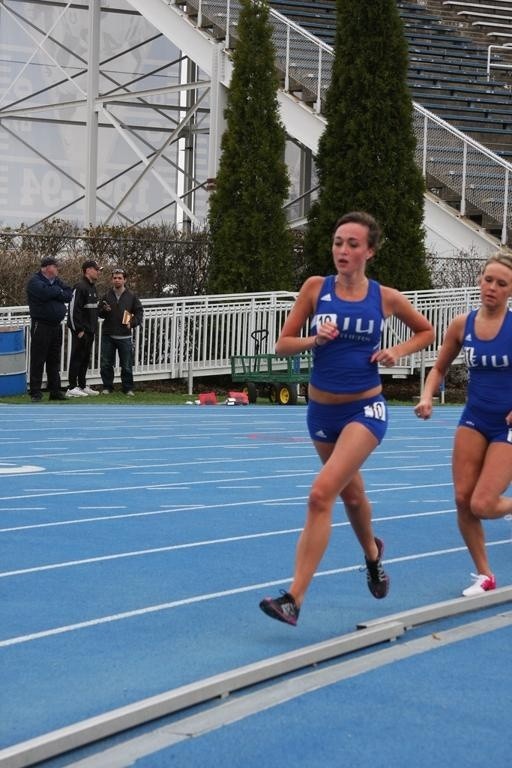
[111,268,125,273]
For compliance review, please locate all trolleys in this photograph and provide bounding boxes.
[230,328,313,405]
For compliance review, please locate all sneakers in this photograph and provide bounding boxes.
[462,572,497,599]
[101,388,110,395]
[49,391,69,400]
[125,390,136,397]
[79,385,100,397]
[364,537,390,601]
[259,592,300,629]
[30,393,44,402]
[64,386,88,398]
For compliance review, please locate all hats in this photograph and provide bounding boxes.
[82,260,104,271]
[40,256,58,266]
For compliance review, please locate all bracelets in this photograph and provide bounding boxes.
[314,336,323,347]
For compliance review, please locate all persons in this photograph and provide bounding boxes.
[98,268,142,398]
[27,258,73,403]
[65,261,104,398]
[414,252,512,598]
[259,212,436,626]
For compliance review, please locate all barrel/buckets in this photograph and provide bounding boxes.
[0,329,27,397]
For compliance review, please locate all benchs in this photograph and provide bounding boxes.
[182,1,512,250]
